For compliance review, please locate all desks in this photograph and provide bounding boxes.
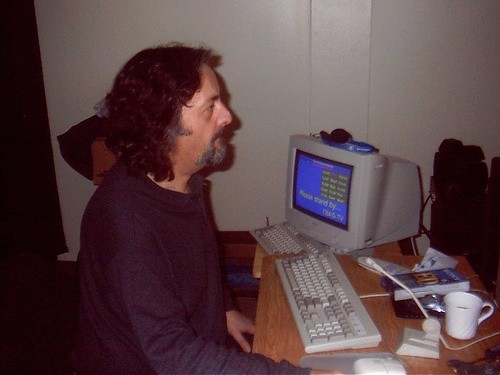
[253,227,500,375]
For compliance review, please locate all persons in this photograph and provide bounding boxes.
[71,44,361,375]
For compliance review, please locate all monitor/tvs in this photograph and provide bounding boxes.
[285,133,420,251]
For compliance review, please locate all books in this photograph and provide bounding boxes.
[381,267,469,302]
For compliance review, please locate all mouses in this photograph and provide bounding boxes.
[353,356,406,375]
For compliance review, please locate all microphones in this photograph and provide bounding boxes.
[365,257,440,359]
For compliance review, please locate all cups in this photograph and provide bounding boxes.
[444,292,494,340]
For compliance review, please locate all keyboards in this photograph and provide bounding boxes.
[274,251,381,354]
[249,222,320,258]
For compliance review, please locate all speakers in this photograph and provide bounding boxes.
[430,144,489,258]
